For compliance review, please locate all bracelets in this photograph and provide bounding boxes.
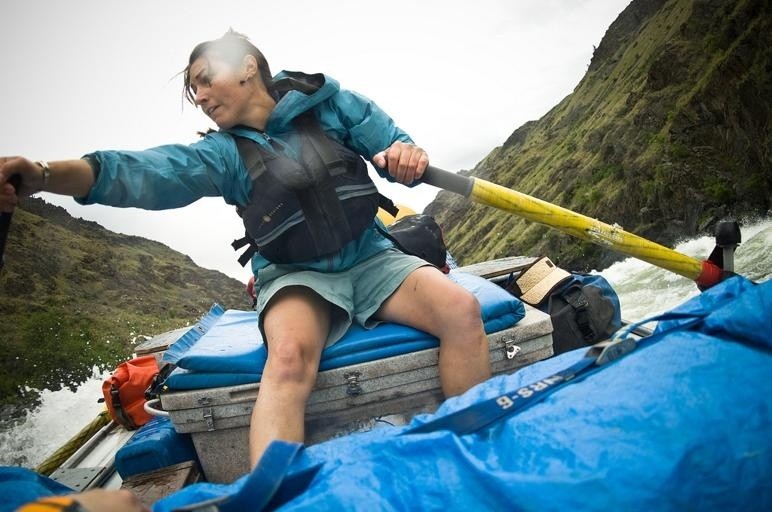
[35,160,50,192]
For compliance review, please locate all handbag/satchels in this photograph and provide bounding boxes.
[102,355,162,432]
[540,269,623,357]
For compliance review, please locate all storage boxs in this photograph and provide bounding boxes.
[160,298,554,486]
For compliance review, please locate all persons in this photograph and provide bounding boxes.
[0,26,491,474]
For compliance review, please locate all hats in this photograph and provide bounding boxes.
[508,255,576,307]
